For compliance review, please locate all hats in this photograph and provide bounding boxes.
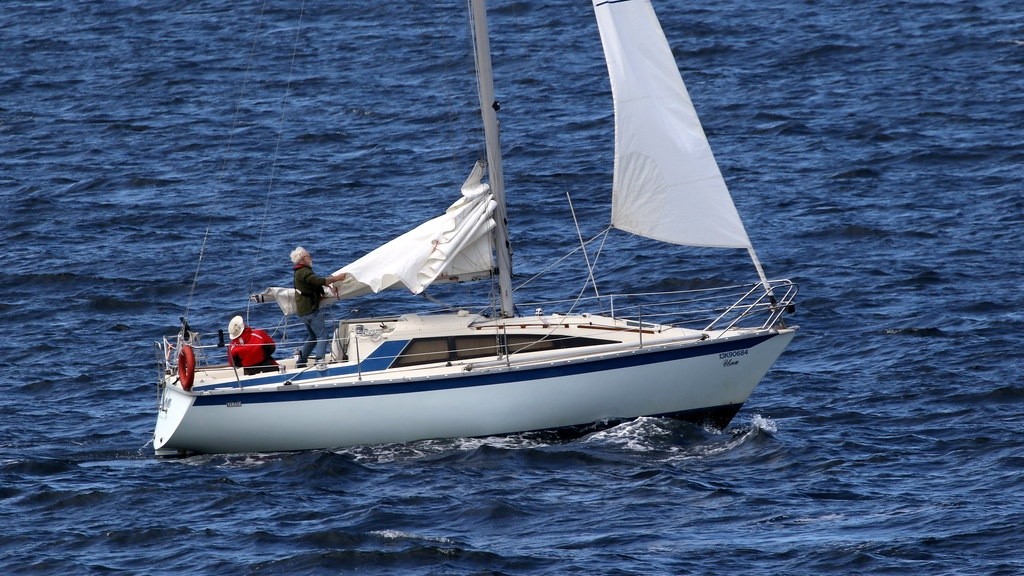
[227,315,244,340]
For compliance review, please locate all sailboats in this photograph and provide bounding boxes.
[151,0,800,457]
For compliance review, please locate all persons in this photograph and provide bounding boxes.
[227,315,279,375]
[290,246,346,369]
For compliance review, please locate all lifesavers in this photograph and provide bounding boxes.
[178,345,196,391]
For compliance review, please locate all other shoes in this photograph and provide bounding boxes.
[295,360,308,368]
[315,359,326,365]
